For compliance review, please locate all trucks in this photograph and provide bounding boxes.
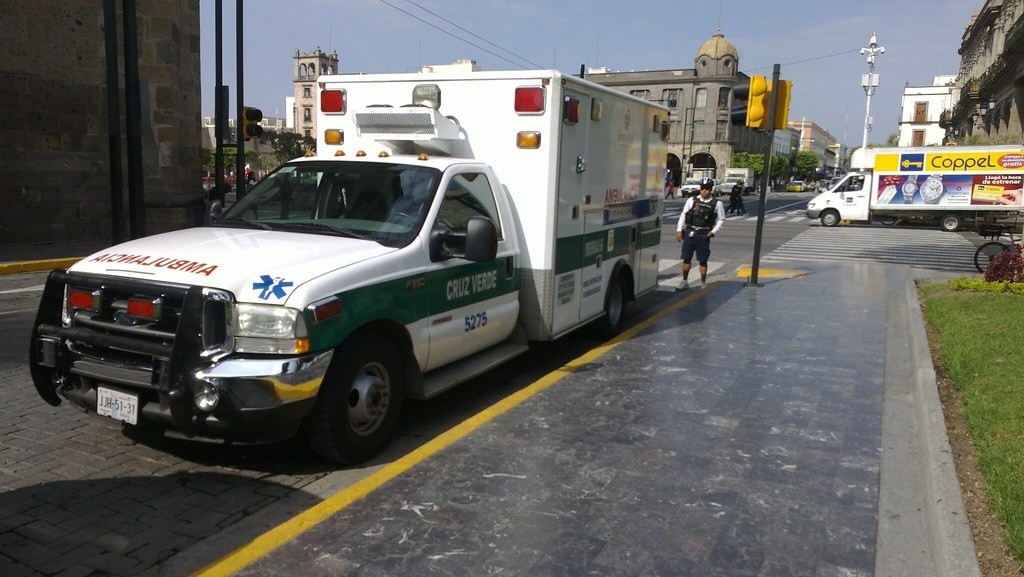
[804,144,1024,232]
[720,167,755,197]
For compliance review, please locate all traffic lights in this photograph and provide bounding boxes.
[731,74,767,129]
[242,105,264,139]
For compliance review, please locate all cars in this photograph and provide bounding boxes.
[681,177,722,198]
[786,177,843,192]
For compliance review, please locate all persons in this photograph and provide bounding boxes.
[247,171,259,220]
[854,180,862,190]
[665,179,675,199]
[675,177,725,292]
[208,182,233,223]
[810,179,816,192]
[725,180,747,215]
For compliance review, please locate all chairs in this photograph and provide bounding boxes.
[347,190,388,222]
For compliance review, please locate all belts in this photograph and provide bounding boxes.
[687,226,710,230]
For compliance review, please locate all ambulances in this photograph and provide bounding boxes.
[28,56,672,469]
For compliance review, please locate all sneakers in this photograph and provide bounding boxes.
[676,280,689,291]
[701,282,706,289]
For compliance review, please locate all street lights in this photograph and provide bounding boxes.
[858,32,886,181]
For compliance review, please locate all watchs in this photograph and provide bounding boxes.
[902,175,919,204]
[920,174,944,203]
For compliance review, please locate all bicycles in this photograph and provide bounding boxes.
[974,218,1024,274]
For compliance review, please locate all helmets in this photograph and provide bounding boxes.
[736,178,744,184]
[700,177,714,186]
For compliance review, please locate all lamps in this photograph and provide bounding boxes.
[949,94,997,136]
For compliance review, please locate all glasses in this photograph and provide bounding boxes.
[701,186,711,190]
